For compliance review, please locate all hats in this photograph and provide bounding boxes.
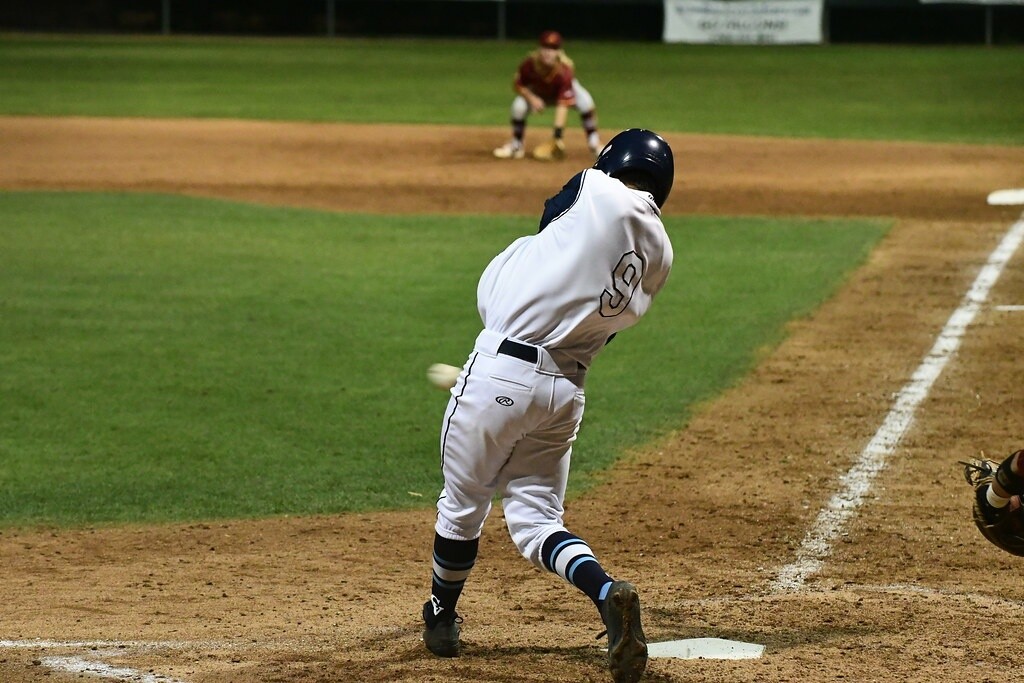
[537,31,560,49]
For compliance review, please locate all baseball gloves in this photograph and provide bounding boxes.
[964,456,1003,489]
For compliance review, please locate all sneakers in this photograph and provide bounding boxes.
[587,132,605,158]
[595,581,648,683]
[423,600,463,658]
[493,136,524,159]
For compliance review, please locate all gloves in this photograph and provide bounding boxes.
[530,97,545,116]
[551,138,565,160]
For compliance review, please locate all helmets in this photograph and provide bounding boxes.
[592,128,674,209]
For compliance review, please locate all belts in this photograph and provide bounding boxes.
[497,339,586,369]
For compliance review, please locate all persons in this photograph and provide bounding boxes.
[422,127,675,683]
[492,29,605,160]
[958,449,1024,557]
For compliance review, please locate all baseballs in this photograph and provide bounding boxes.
[427,363,464,390]
